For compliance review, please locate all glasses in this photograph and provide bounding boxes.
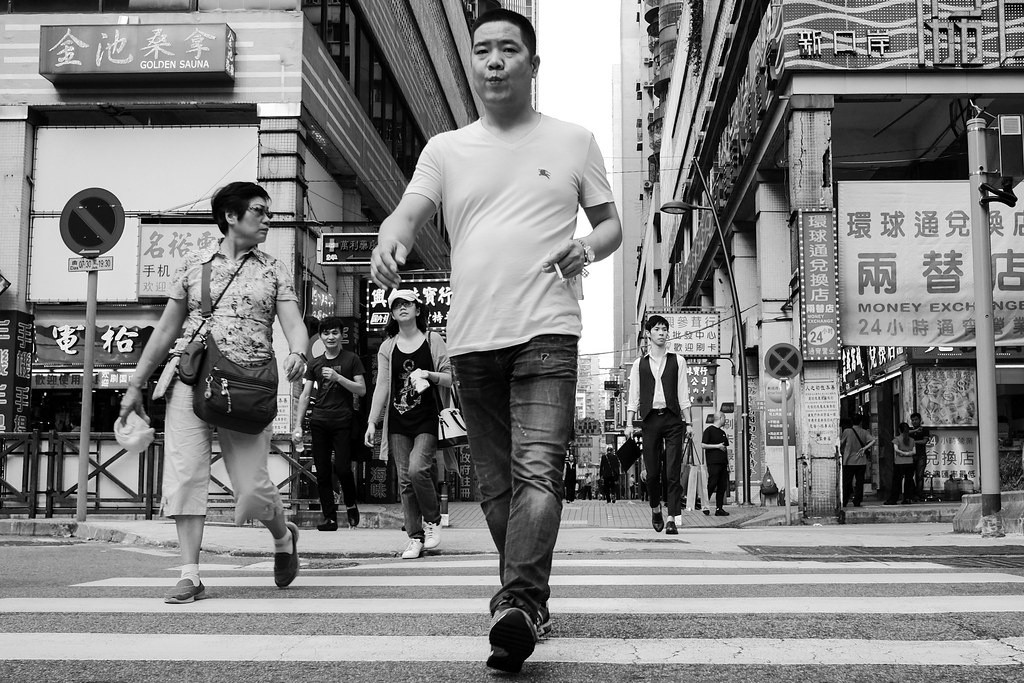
[393,301,415,311]
[246,205,274,220]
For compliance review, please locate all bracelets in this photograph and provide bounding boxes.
[726,466,729,467]
[686,423,693,427]
[625,425,633,428]
[128,381,142,389]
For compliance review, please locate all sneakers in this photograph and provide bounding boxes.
[422,521,441,548]
[402,538,426,559]
[703,510,710,515]
[715,508,729,516]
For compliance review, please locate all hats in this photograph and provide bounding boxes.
[387,288,422,310]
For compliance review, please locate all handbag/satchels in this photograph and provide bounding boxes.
[866,450,872,462]
[193,332,279,435]
[779,486,799,505]
[680,438,711,513]
[437,365,467,440]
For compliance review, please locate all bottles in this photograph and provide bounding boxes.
[958,474,974,501]
[294,433,304,453]
[944,474,959,501]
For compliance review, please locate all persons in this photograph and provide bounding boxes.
[70,421,81,432]
[291,316,366,531]
[629,474,636,500]
[582,474,592,500]
[624,315,695,534]
[562,454,578,503]
[364,289,452,558]
[840,413,930,507]
[701,411,729,516]
[119,181,311,603]
[640,478,649,501]
[593,447,619,503]
[370,9,623,674]
[621,485,625,499]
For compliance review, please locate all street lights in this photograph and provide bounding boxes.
[660,157,751,505]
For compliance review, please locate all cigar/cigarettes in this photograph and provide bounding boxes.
[554,262,563,279]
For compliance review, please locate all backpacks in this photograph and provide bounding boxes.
[761,467,779,495]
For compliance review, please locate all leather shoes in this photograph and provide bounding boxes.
[667,521,680,534]
[651,503,664,533]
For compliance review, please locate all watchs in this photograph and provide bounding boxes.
[574,239,595,266]
[289,352,308,363]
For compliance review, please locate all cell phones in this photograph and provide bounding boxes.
[410,367,430,395]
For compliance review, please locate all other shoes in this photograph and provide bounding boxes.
[165,578,206,604]
[486,607,537,673]
[274,521,300,586]
[346,501,360,525]
[840,494,925,507]
[317,519,338,531]
[532,608,552,638]
[563,489,650,503]
